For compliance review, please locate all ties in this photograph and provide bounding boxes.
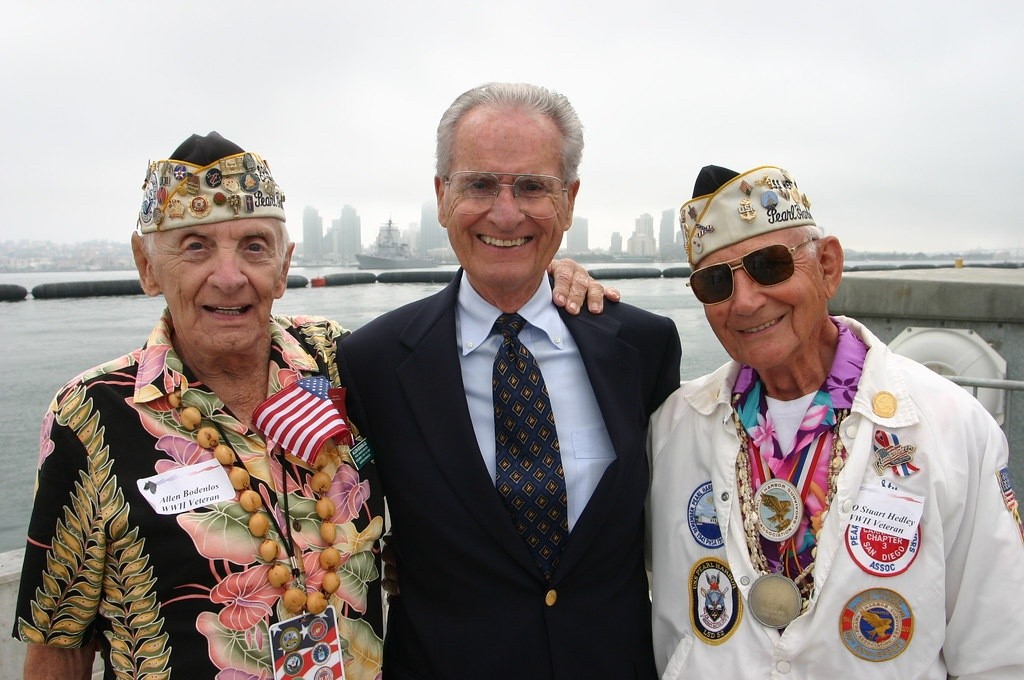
[490,311,570,583]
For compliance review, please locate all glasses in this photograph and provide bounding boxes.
[444,170,570,220]
[686,237,818,306]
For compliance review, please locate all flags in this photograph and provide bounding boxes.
[250,374,350,464]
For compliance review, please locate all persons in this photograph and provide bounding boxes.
[11,130,620,679]
[645,165,1023,680]
[336,82,684,680]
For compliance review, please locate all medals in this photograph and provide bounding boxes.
[747,572,803,630]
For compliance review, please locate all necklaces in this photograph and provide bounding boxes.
[168,386,341,616]
[733,391,848,594]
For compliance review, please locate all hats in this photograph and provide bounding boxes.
[678,164,816,272]
[136,131,287,234]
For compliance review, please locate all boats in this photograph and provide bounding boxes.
[353,218,438,269]
